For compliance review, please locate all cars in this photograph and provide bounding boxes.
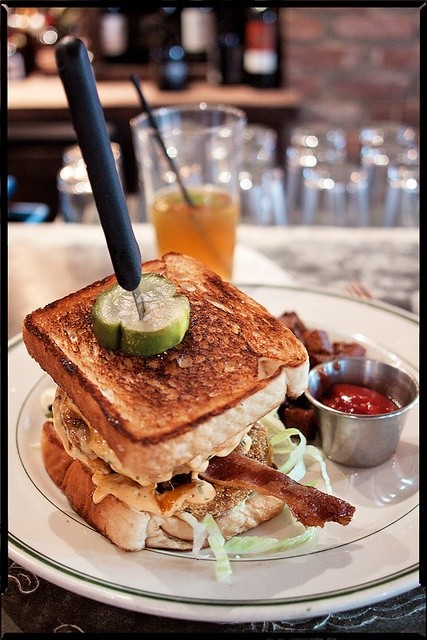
[243,162,286,232]
[293,125,346,213]
[384,164,422,227]
[240,125,276,169]
[301,164,371,228]
[129,104,246,287]
[305,355,419,468]
[362,123,415,152]
[363,147,419,224]
[59,164,111,227]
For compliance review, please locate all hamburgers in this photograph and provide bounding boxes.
[22,251,351,556]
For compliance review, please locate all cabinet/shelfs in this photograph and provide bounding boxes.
[242,5,282,89]
[218,2,244,84]
[97,7,128,59]
[153,6,188,89]
[180,6,217,80]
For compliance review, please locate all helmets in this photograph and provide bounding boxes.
[8,283,422,626]
